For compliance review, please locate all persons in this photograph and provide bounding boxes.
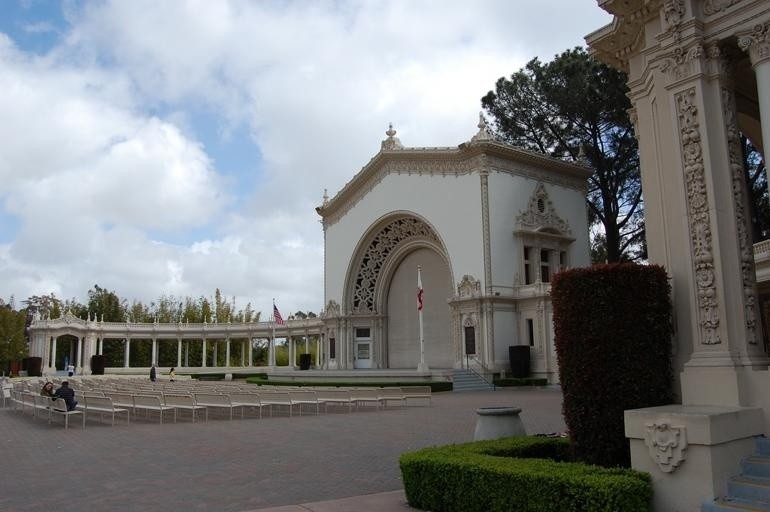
[53,382,78,412]
[169,368,176,383]
[150,366,157,381]
[64,352,68,371]
[41,382,61,409]
[67,363,75,376]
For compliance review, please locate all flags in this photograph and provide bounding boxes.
[274,304,284,324]
[417,272,423,310]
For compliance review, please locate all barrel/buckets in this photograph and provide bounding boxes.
[27,356,41,377]
[92,354,105,375]
[299,353,312,371]
[472,404,524,442]
[509,345,531,378]
